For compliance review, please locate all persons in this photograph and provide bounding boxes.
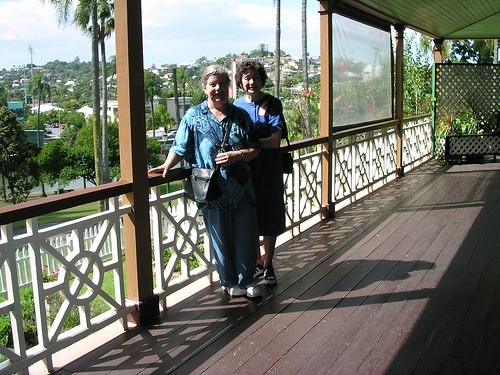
[233,60,286,284]
[148,63,263,298]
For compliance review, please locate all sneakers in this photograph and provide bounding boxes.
[222,284,246,296]
[263,267,276,284]
[254,262,263,278]
[238,280,261,297]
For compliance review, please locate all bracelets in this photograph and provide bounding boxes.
[240,150,245,161]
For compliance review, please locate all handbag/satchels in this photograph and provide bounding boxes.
[184,168,218,203]
[280,147,293,174]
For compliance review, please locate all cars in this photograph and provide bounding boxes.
[45,128,52,134]
